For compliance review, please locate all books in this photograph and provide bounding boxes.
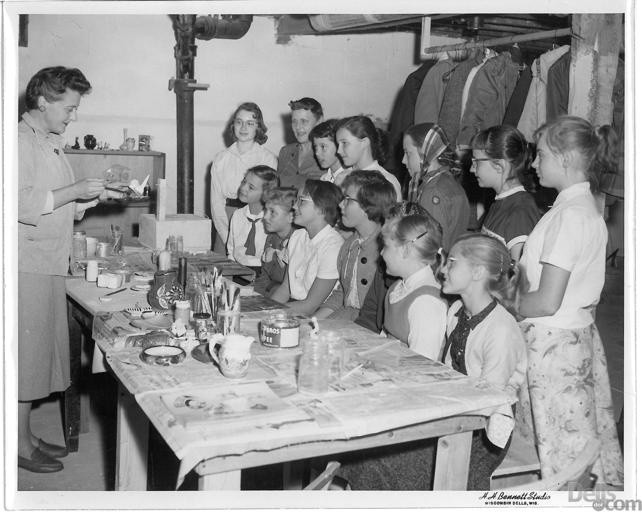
[162,381,313,431]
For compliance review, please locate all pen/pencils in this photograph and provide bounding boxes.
[216,283,240,334]
[341,363,364,379]
[195,266,224,322]
[110,224,123,255]
[104,287,127,296]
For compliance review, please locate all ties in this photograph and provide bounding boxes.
[245,217,261,255]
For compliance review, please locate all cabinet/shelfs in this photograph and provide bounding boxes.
[62,150,167,247]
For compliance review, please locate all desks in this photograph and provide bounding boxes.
[101,317,487,490]
[62,274,293,488]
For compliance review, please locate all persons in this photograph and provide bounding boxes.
[270,178,345,317]
[380,215,451,362]
[329,237,530,491]
[354,202,448,334]
[470,125,543,263]
[334,116,403,202]
[313,170,396,320]
[18,66,111,473]
[247,187,298,298]
[308,119,352,183]
[402,123,471,253]
[210,102,278,256]
[502,116,624,491]
[226,165,281,286]
[278,97,327,187]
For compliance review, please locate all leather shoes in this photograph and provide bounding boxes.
[35,438,70,458]
[19,447,63,474]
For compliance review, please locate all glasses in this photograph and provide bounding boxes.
[235,118,259,130]
[340,194,360,206]
[447,256,471,269]
[293,196,313,205]
[471,157,492,168]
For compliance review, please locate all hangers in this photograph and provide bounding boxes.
[422,28,572,59]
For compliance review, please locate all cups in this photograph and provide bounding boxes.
[297,339,330,398]
[316,329,346,392]
[167,234,183,261]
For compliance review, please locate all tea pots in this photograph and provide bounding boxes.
[207,333,255,379]
